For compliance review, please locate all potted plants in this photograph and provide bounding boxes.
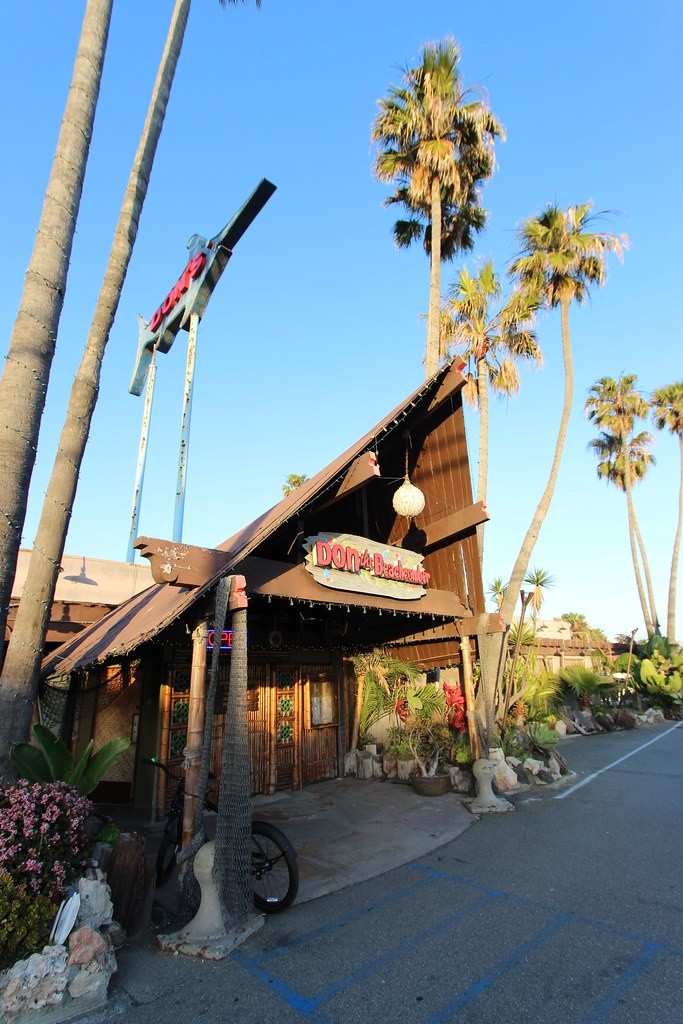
[9,724,131,839]
[351,651,477,797]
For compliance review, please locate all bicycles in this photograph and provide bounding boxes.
[136,754,302,915]
